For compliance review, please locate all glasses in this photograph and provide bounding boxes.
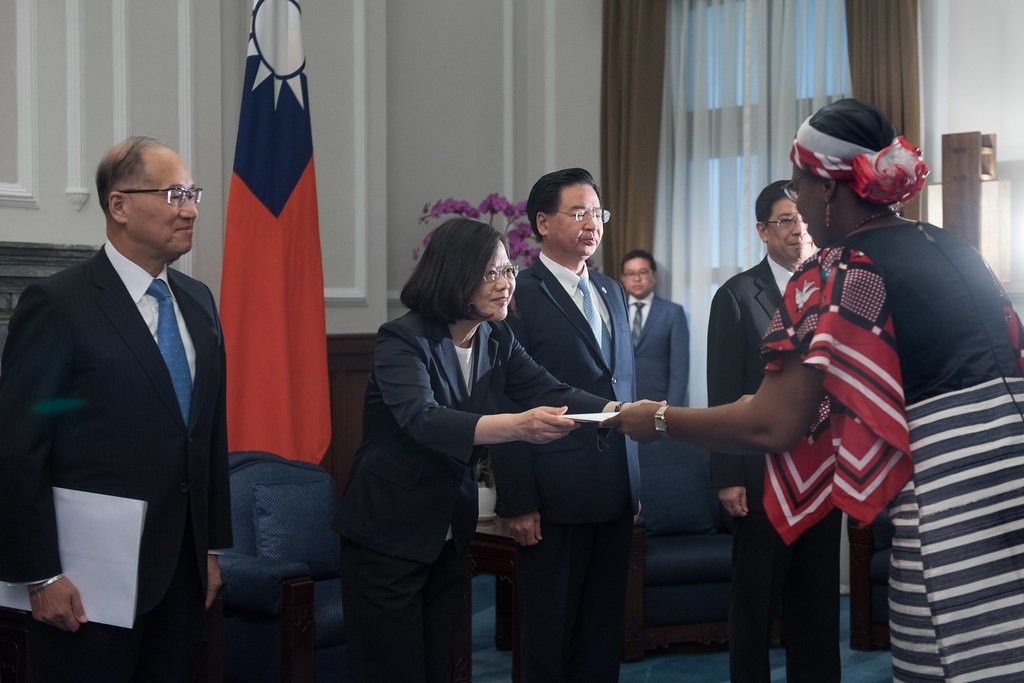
[764,217,804,228]
[781,171,809,203]
[481,265,519,281]
[105,185,201,207]
[548,208,611,224]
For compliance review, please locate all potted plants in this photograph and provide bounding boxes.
[475,451,497,521]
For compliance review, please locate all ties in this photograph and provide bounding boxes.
[146,280,192,429]
[577,279,614,372]
[630,302,644,346]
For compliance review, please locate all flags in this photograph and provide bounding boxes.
[217,0,333,466]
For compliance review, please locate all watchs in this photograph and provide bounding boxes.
[653,405,671,441]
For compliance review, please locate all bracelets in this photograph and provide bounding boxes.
[25,573,66,598]
[613,401,628,413]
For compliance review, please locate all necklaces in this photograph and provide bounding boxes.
[854,211,901,231]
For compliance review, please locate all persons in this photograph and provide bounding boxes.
[615,248,688,408]
[708,181,844,683]
[0,134,234,683]
[331,217,667,683]
[487,168,643,683]
[598,99,1024,683]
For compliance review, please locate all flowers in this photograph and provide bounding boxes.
[412,192,601,272]
[850,135,928,208]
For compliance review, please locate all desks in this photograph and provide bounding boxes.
[473,520,521,648]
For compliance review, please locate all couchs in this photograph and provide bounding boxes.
[207,451,347,683]
[620,436,782,664]
